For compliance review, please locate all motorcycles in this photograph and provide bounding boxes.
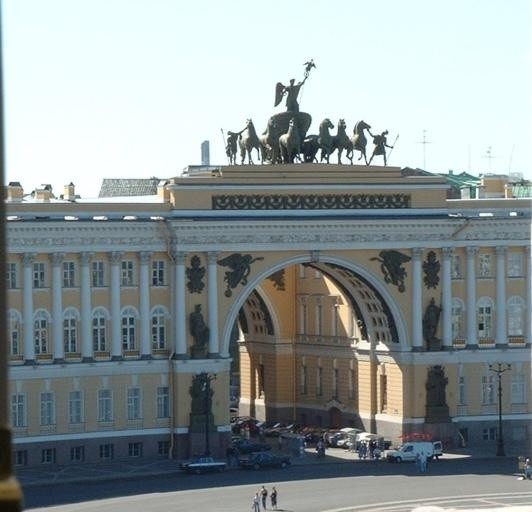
[316,448,326,460]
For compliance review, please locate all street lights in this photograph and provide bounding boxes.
[194,369,225,458]
[489,362,512,456]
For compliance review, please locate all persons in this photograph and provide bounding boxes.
[282,78,301,112]
[359,439,381,461]
[367,128,394,167]
[415,450,431,474]
[253,485,278,512]
[188,304,207,346]
[424,298,439,338]
[317,439,325,455]
[227,131,243,166]
[523,459,531,479]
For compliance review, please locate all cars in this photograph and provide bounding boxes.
[179,457,225,474]
[228,395,393,453]
[237,452,291,470]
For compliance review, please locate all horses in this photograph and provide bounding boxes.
[279,118,301,164]
[347,120,371,166]
[330,119,350,165]
[258,119,277,165]
[240,118,259,165]
[306,118,334,164]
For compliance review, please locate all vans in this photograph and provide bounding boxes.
[387,441,443,463]
